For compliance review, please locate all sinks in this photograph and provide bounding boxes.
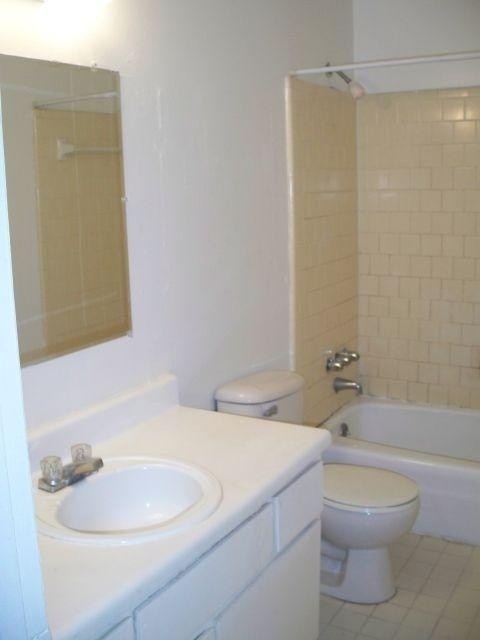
[29,454,223,547]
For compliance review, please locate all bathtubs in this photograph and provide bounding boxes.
[317,398,480,546]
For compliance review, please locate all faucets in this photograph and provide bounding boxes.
[36,443,103,493]
[332,376,363,395]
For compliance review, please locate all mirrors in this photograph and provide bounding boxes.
[0,49,135,370]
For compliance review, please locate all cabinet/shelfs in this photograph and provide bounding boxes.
[102,459,325,640]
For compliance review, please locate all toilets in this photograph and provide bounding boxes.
[215,371,422,606]
